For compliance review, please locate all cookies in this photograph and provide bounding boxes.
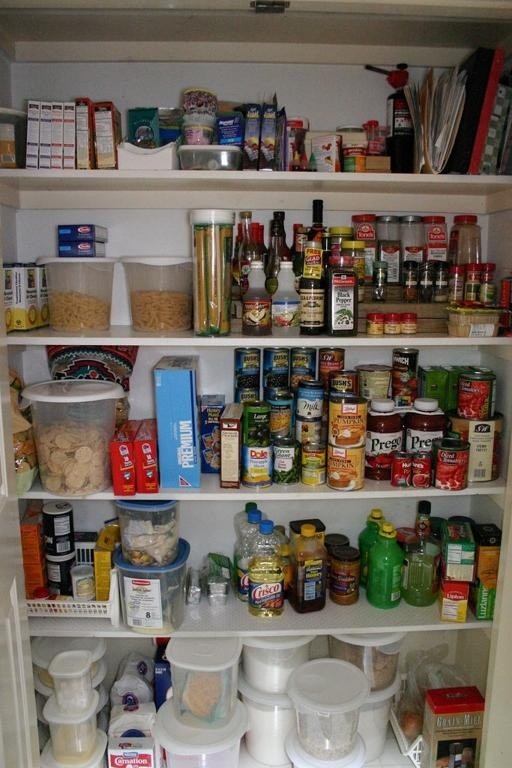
[36,423,112,499]
[9,414,35,474]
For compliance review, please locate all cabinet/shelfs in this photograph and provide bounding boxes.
[0,0,511,768]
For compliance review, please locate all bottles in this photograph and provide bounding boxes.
[404,398,447,456]
[244,521,290,619]
[236,200,511,337]
[232,503,259,586]
[234,511,263,602]
[42,502,76,599]
[366,399,404,481]
[403,501,443,607]
[367,525,403,610]
[358,510,385,591]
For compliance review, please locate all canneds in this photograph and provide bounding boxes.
[443,362,471,412]
[267,399,295,444]
[328,371,360,401]
[318,347,345,396]
[263,348,290,400]
[354,365,392,400]
[297,382,324,422]
[390,452,413,487]
[457,372,497,419]
[414,455,432,488]
[233,348,261,402]
[392,348,420,403]
[330,546,360,606]
[291,525,325,613]
[470,364,497,419]
[419,364,448,414]
[296,420,324,450]
[290,345,317,400]
[431,438,471,491]
[303,446,327,469]
[243,445,273,489]
[324,535,350,589]
[326,444,365,491]
[243,404,269,446]
[300,467,326,485]
[326,398,367,447]
[448,410,504,483]
[274,442,299,485]
